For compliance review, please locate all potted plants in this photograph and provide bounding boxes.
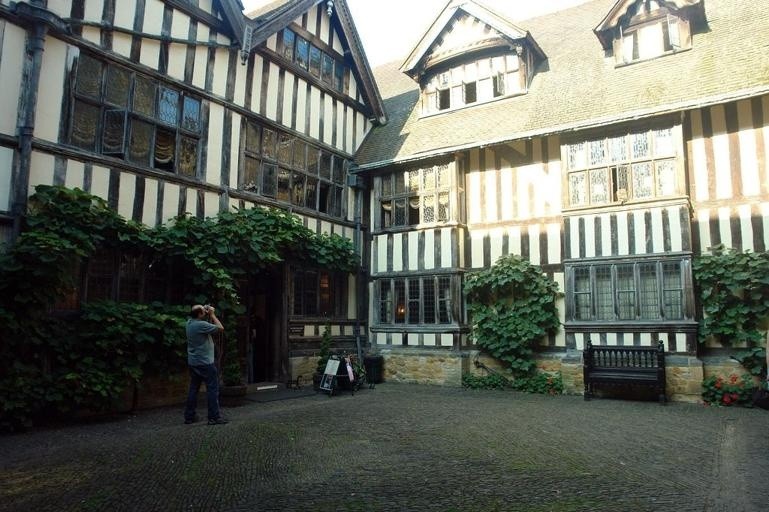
[313,322,335,394]
[219,313,248,408]
[63,358,136,420]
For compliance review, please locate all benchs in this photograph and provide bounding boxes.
[581,339,667,404]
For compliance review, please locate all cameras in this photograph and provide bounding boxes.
[204,303,211,310]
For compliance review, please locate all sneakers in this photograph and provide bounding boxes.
[208,418,230,425]
[185,416,199,424]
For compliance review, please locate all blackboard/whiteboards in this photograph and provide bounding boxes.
[318,354,343,392]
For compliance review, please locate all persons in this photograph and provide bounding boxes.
[183,303,230,425]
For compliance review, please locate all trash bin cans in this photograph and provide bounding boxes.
[364,354,383,384]
